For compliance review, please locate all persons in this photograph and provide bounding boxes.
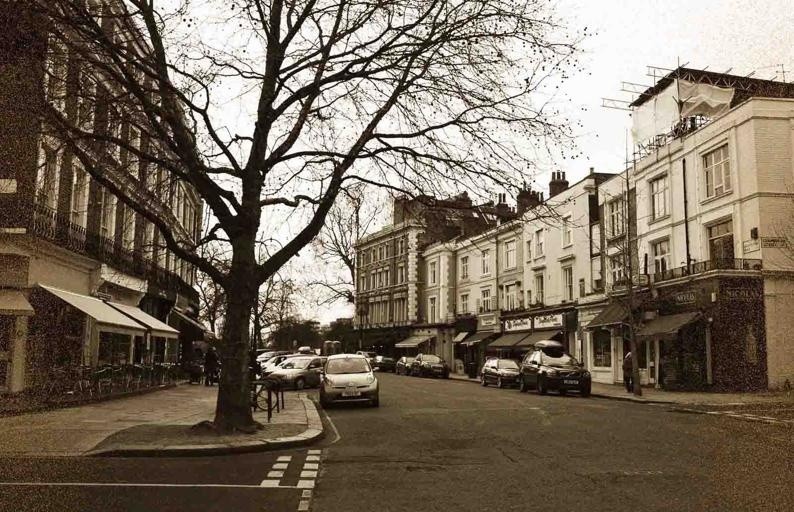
[621,352,634,393]
[203,345,218,387]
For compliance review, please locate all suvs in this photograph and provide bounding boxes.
[516,341,593,397]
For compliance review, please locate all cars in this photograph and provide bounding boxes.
[252,344,449,391]
[314,348,381,407]
[478,356,520,390]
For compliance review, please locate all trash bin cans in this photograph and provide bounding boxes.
[468,362,477,379]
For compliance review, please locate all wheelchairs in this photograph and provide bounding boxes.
[207,359,222,385]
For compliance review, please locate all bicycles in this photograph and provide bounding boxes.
[245,366,277,412]
